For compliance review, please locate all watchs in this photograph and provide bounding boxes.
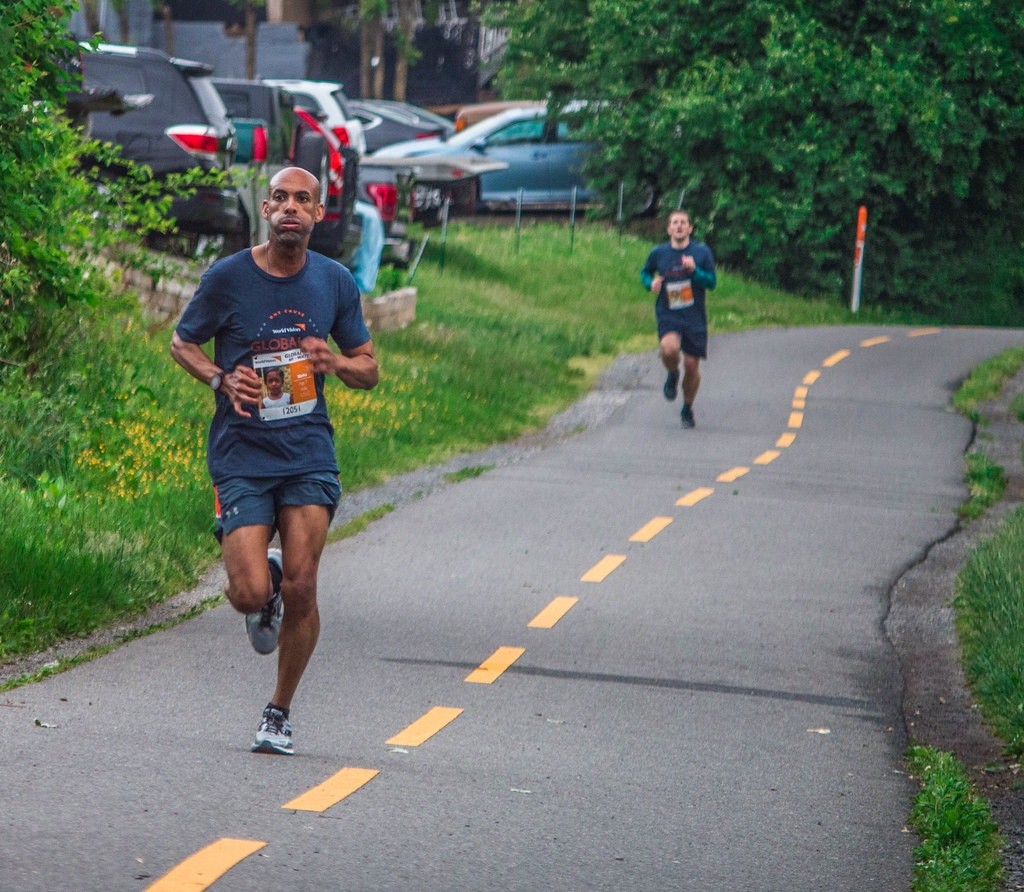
[211,371,228,389]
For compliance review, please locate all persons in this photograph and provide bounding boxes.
[640,209,717,425]
[171,166,379,754]
[260,366,293,410]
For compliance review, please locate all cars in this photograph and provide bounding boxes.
[262,79,363,155]
[348,97,661,214]
[60,37,239,232]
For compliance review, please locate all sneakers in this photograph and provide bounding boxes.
[663,368,680,401]
[250,703,294,755]
[680,408,695,429]
[246,548,284,655]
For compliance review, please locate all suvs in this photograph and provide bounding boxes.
[184,78,359,265]
